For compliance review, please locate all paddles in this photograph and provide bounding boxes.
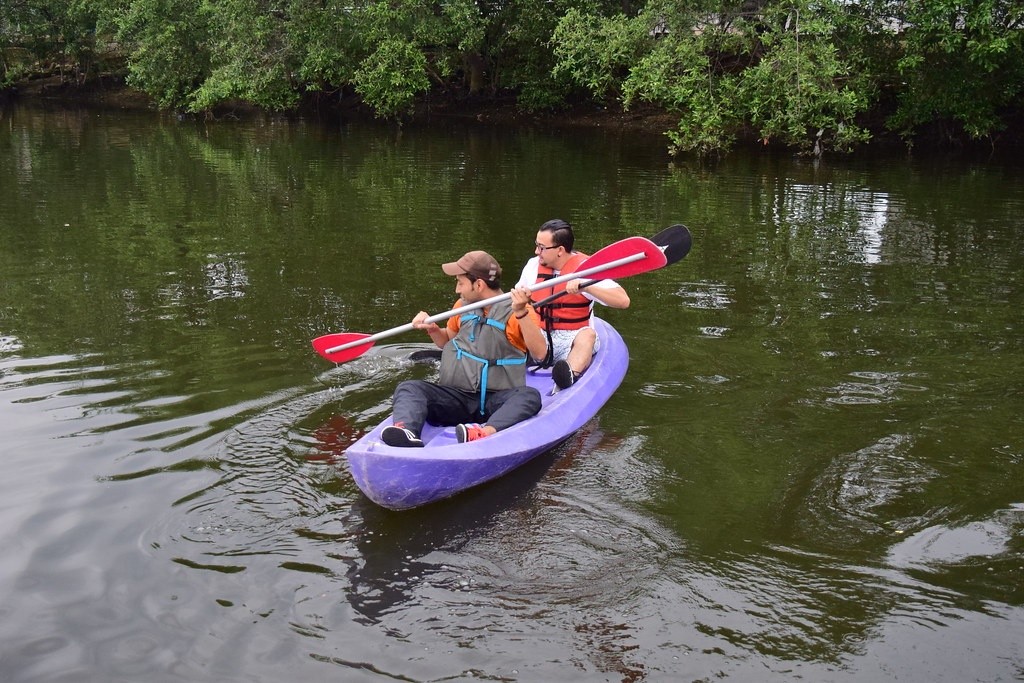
[309,237,668,365]
[529,220,696,311]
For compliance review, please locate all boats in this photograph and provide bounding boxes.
[344,315,630,513]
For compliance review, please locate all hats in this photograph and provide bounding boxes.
[442,251,502,283]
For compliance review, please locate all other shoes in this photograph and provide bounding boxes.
[551,360,575,390]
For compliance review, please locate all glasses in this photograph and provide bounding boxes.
[534,241,565,252]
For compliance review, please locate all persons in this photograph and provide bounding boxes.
[515,219,631,396]
[381,250,547,447]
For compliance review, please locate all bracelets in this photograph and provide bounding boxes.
[515,309,529,319]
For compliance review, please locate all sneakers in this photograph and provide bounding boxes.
[455,423,486,442]
[381,422,425,447]
